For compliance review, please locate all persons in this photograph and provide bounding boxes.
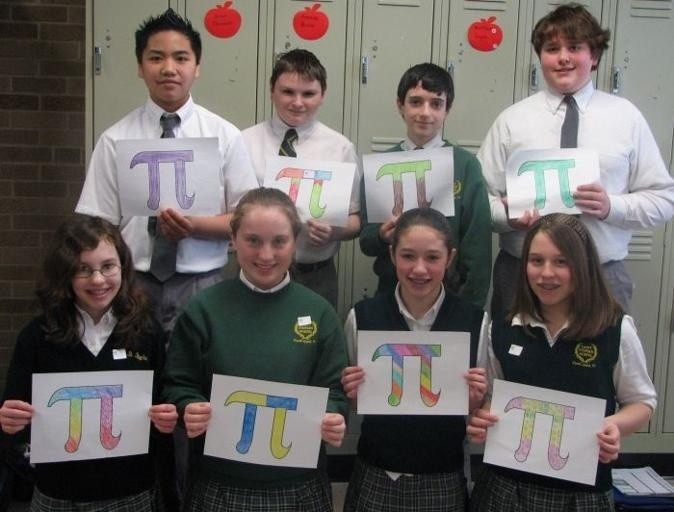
[0,215,178,511]
[75,5,264,496]
[461,212,658,512]
[474,1,673,319]
[338,208,490,511]
[360,61,492,313]
[240,48,361,319]
[161,184,351,512]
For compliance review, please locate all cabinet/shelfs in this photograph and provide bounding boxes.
[617,217,673,453]
[83,1,178,182]
[335,239,376,326]
[355,1,442,172]
[527,2,610,97]
[179,1,267,131]
[442,1,528,156]
[611,1,674,176]
[269,0,355,143]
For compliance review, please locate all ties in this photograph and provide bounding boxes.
[150,115,178,283]
[558,96,580,149]
[277,128,298,159]
